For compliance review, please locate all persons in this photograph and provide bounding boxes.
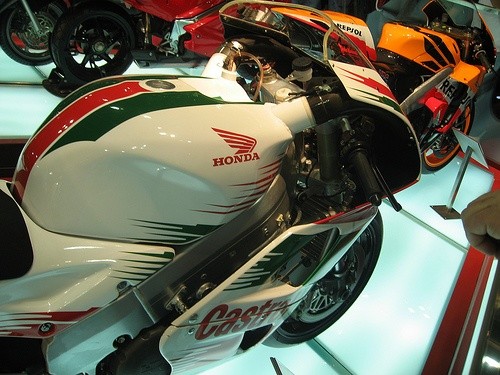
[460,188,500,262]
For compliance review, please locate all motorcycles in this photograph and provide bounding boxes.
[0,0,67,66]
[219,0,500,173]
[50,2,289,86]
[1,0,422,375]
